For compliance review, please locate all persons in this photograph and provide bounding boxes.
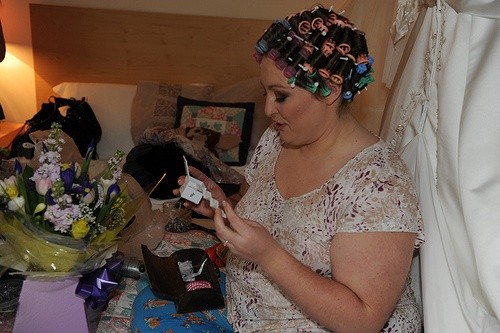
[172,7,428,333]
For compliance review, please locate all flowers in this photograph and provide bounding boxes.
[0,122,151,333]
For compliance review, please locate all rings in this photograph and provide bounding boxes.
[222,240,228,250]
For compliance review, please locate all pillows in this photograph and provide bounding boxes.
[129,82,273,166]
